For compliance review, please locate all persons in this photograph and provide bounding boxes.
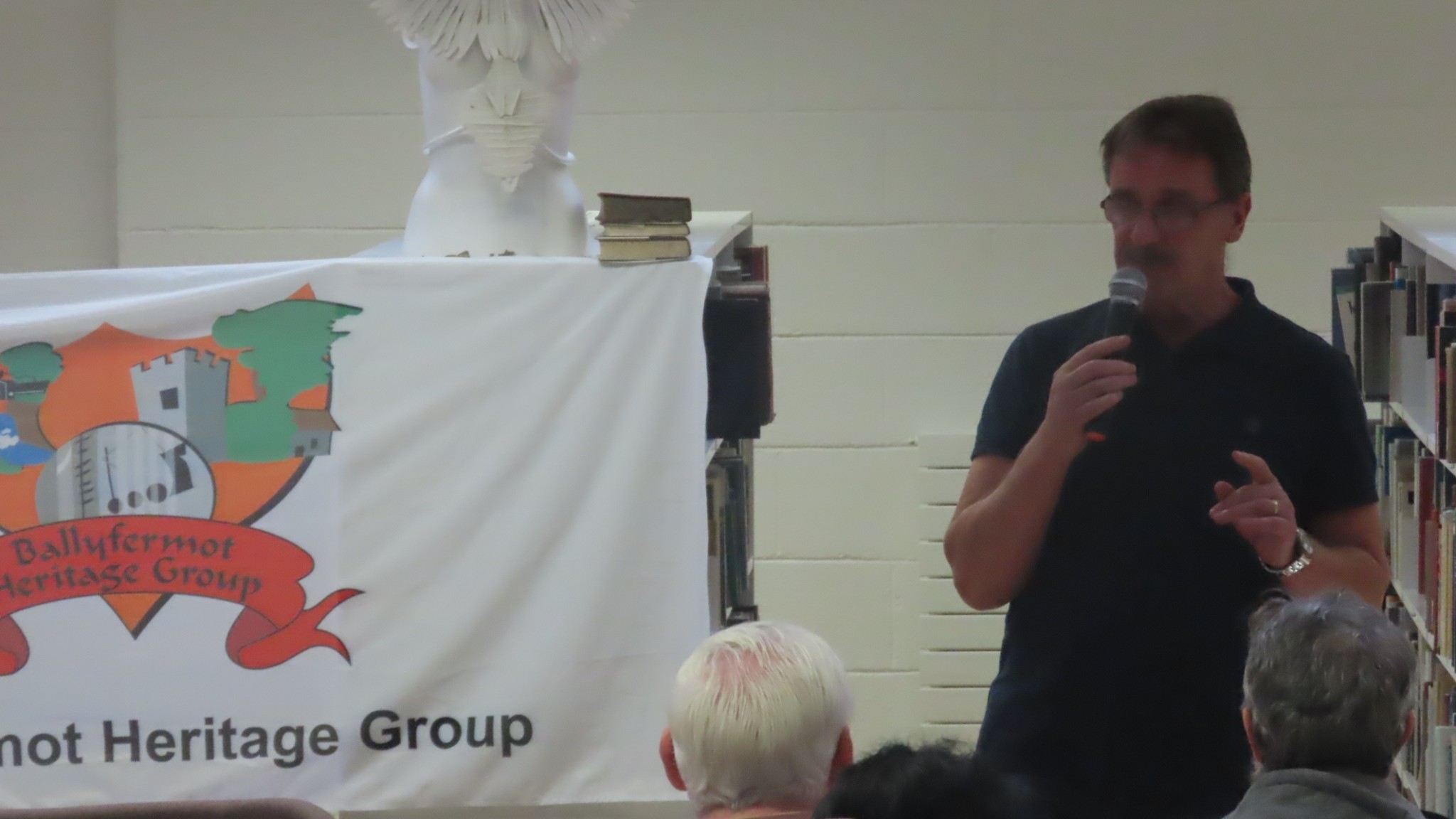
[656,620,857,819]
[945,96,1394,818]
[1223,588,1435,819]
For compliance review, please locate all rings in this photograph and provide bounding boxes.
[1271,499,1279,517]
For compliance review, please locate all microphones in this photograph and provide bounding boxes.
[1079,265,1148,443]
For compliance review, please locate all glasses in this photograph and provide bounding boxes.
[1101,190,1228,231]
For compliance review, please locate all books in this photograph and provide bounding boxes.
[1329,233,1456,819]
[594,190,693,264]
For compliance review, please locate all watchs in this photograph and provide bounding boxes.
[1257,523,1314,579]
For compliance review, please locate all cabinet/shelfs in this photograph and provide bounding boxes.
[1375,204,1456,819]
